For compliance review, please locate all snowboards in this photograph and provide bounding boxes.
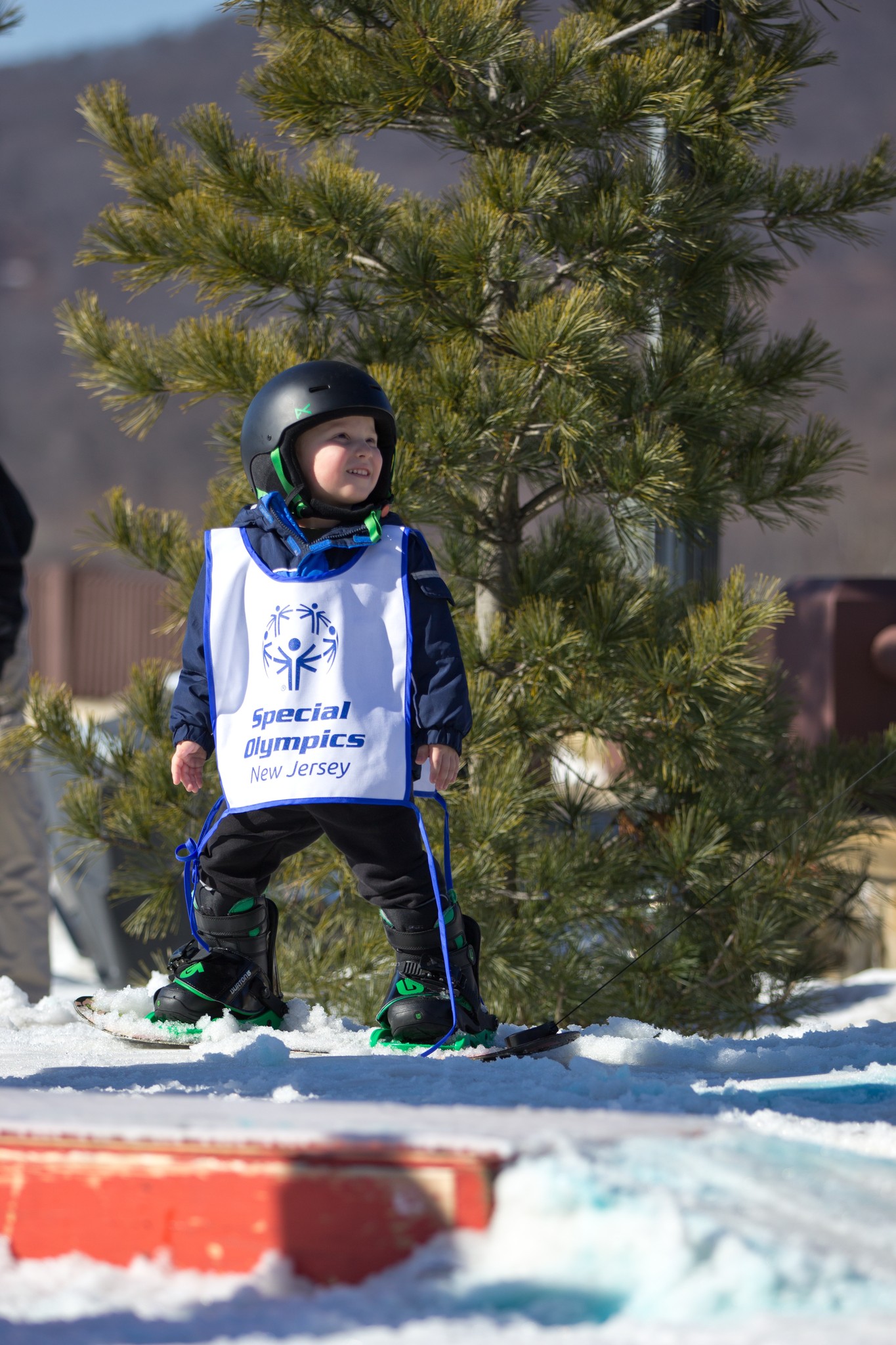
[73,996,582,1064]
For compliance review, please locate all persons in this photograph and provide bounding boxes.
[0,460,39,685]
[153,359,480,1043]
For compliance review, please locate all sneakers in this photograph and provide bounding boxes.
[144,896,289,1040]
[368,890,501,1052]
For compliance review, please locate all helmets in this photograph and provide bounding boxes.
[239,360,401,518]
